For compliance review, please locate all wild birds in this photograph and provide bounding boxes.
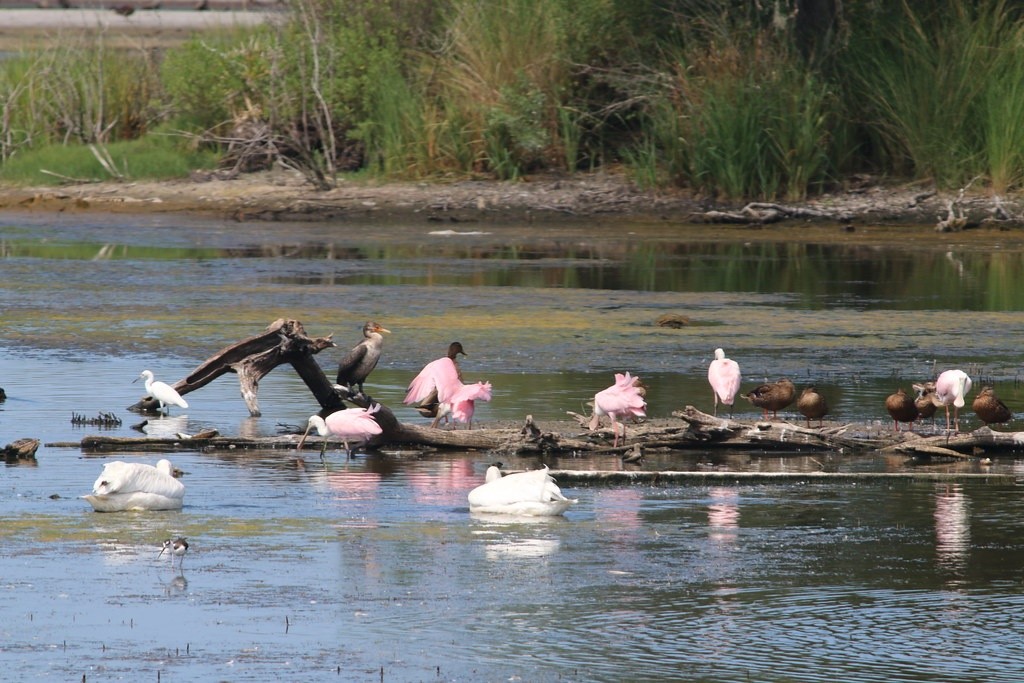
[972,386,1012,431]
[132,369,189,412]
[709,347,742,418]
[296,403,383,459]
[739,379,798,421]
[935,369,972,431]
[337,320,391,392]
[913,381,942,430]
[594,370,648,447]
[415,342,468,428]
[798,386,828,427]
[467,463,579,516]
[401,356,493,429]
[157,537,190,566]
[81,458,186,513]
[886,388,919,431]
